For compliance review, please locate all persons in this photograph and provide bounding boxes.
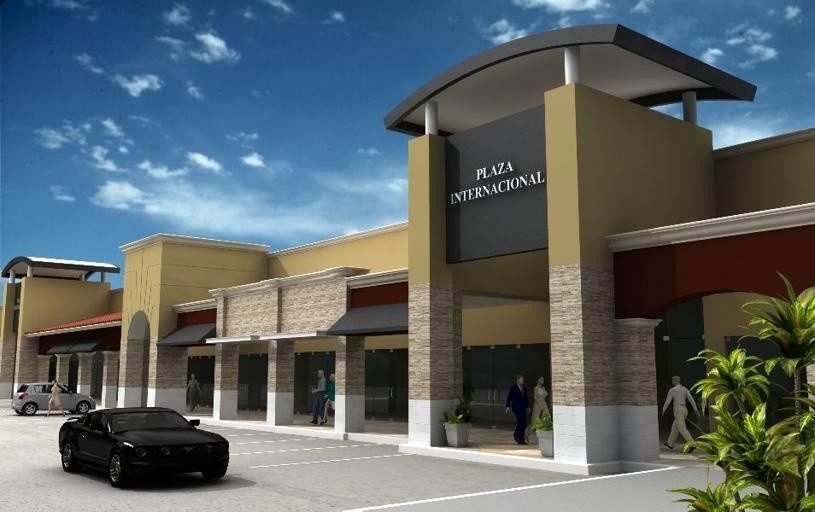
[320,373,335,424]
[526,375,551,443]
[43,378,66,418]
[661,375,701,454]
[186,373,203,411]
[308,369,329,425]
[506,373,531,445]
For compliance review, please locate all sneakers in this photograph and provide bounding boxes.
[687,446,698,454]
[663,442,674,449]
[309,418,327,424]
